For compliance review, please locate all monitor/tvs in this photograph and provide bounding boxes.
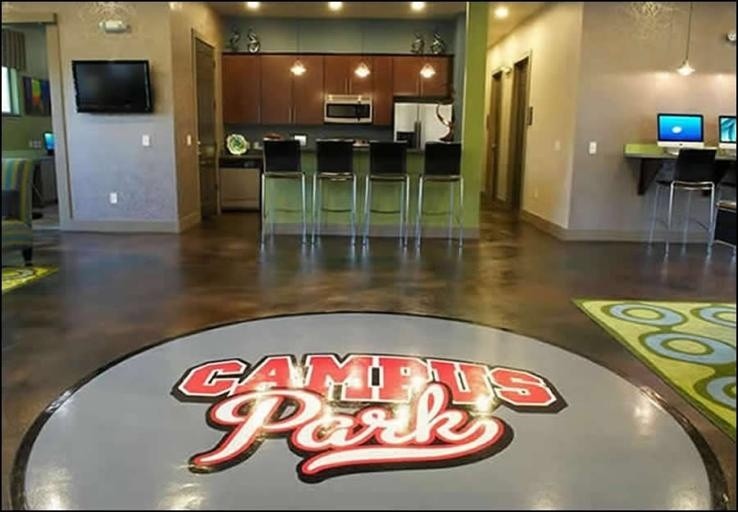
[656,112,705,156]
[42,131,54,156]
[718,114,737,150]
[71,59,153,114]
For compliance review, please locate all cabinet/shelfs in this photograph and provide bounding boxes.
[222,52,455,128]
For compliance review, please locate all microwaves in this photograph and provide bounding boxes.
[322,94,374,125]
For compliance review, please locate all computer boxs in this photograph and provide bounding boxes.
[714,205,738,246]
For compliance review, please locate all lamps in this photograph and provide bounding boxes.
[99,20,133,33]
[419,1,437,79]
[676,2,697,77]
[289,2,307,76]
[354,2,371,79]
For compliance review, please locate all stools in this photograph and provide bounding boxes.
[713,199,737,254]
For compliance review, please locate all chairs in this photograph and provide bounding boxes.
[648,148,716,259]
[260,138,464,248]
[1,158,35,266]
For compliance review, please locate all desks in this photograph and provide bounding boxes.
[32,156,57,208]
[624,142,737,196]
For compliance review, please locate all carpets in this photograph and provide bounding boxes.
[2,261,60,295]
[570,295,737,446]
